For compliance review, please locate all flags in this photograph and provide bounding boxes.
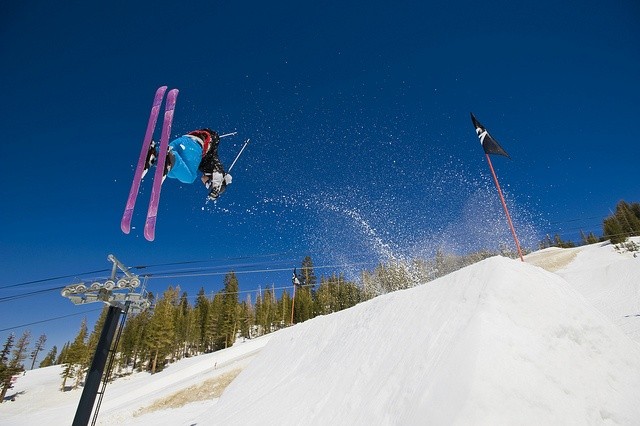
[472,113,514,164]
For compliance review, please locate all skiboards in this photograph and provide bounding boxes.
[121,85,179,243]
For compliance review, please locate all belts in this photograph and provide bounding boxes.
[188,136,203,147]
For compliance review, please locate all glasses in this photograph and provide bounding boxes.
[205,178,212,189]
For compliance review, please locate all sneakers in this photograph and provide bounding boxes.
[163,151,175,175]
[144,145,157,170]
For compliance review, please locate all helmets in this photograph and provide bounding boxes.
[218,179,225,194]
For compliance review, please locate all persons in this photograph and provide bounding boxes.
[144,127,233,201]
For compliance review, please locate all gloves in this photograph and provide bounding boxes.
[209,187,220,198]
